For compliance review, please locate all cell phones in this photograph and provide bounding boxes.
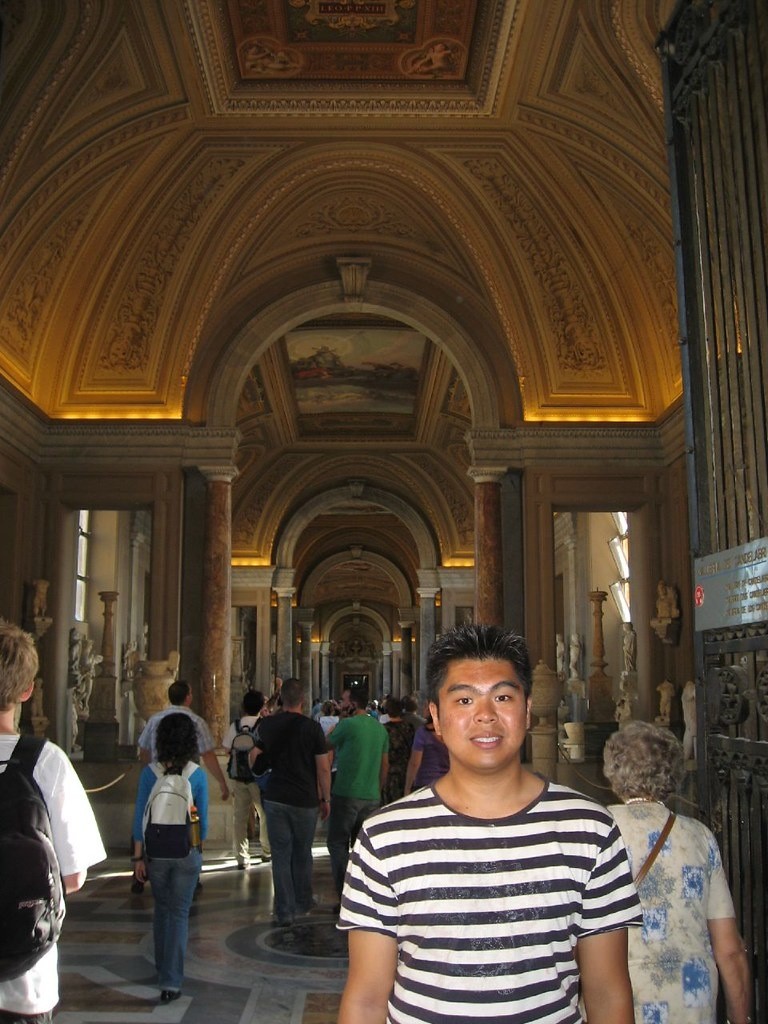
[346,704,356,715]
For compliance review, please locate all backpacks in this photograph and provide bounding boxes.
[141,761,201,862]
[225,717,264,784]
[0,735,68,986]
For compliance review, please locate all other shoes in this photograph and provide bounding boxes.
[196,879,203,889]
[130,871,148,894]
[238,863,250,870]
[262,856,273,862]
[161,990,181,1003]
[332,903,342,914]
[271,901,317,925]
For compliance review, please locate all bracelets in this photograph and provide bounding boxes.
[727,1016,751,1024]
[131,856,143,862]
[322,798,330,803]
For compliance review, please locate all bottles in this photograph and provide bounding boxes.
[189,806,201,846]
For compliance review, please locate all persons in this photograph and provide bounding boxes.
[656,581,674,620]
[622,622,637,671]
[324,684,389,914]
[556,634,564,672]
[79,638,95,712]
[135,713,209,1004]
[130,680,229,902]
[222,691,271,870]
[577,719,752,1024]
[336,618,645,1024]
[0,616,107,1024]
[656,677,675,723]
[247,695,429,841]
[570,632,581,678]
[69,628,83,752]
[143,621,149,661]
[404,696,452,798]
[248,679,331,926]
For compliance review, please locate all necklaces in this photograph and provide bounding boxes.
[625,797,666,809]
